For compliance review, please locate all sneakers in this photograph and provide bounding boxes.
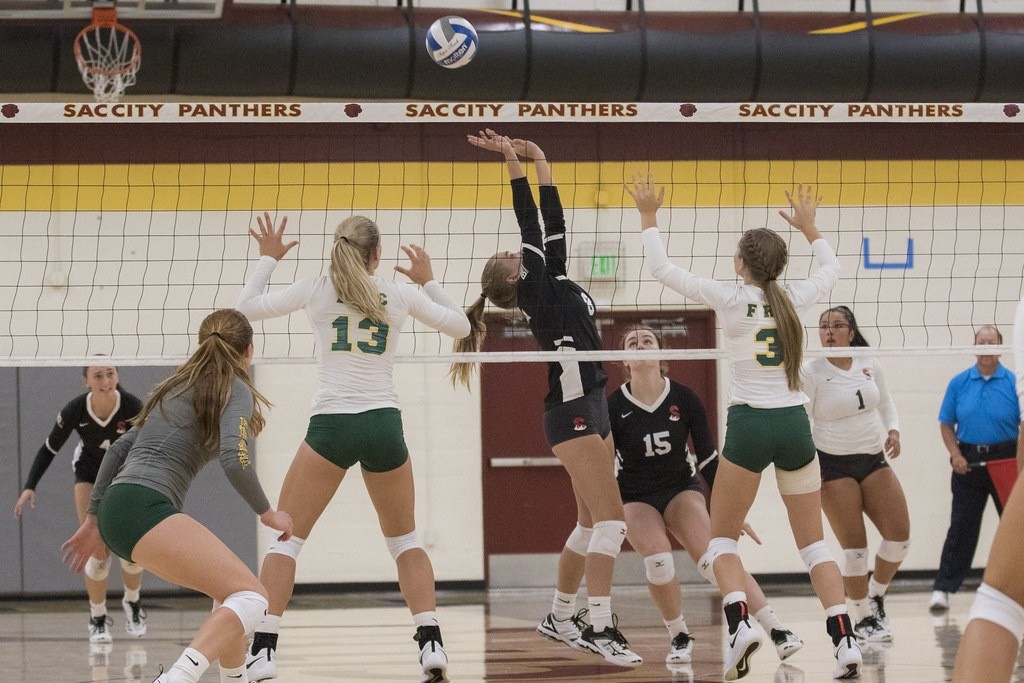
[832,636,864,679]
[88,642,114,666]
[122,598,147,638]
[124,646,147,682]
[853,614,893,642]
[578,613,644,668]
[665,632,695,663]
[88,613,113,643]
[867,594,890,629]
[535,608,590,654]
[770,628,804,660]
[724,619,763,681]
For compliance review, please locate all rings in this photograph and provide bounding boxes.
[955,466,960,471]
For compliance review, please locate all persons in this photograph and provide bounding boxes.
[799,305,910,642]
[449,128,644,667]
[235,212,472,683]
[930,325,1021,610]
[607,326,805,664]
[953,300,1024,683]
[13,354,148,643]
[622,171,863,681]
[60,309,294,683]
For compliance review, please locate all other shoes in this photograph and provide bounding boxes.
[413,625,449,683]
[928,589,949,609]
[150,663,167,683]
[245,637,278,681]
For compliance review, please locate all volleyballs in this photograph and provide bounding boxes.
[425,14,480,69]
